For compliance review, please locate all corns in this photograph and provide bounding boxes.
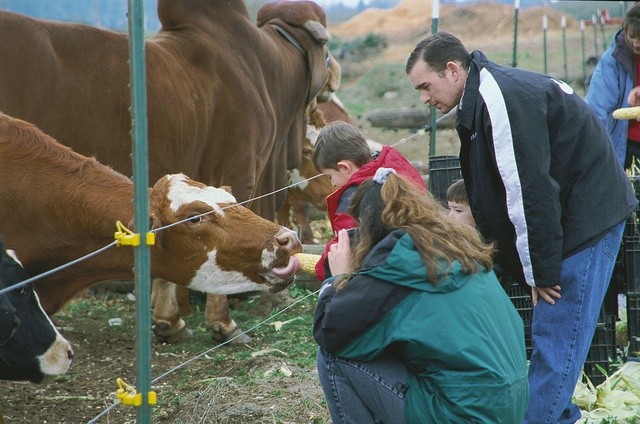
[292,253,322,275]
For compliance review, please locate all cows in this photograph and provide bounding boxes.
[0,112,303,316]
[277,95,382,244]
[0,0,341,344]
[1,248,74,383]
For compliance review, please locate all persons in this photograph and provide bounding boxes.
[583,55,599,87]
[584,1,640,171]
[311,121,433,280]
[627,85,640,123]
[442,179,481,233]
[405,31,637,424]
[310,166,530,423]
